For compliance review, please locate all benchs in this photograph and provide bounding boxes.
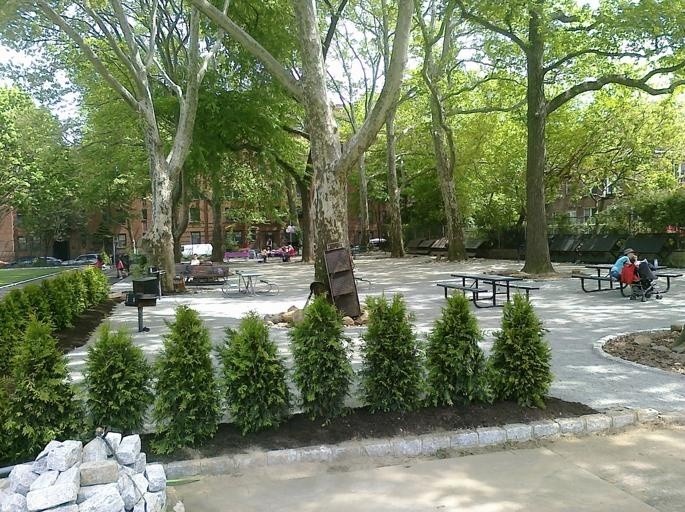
[221,280,241,298]
[572,275,631,297]
[484,281,539,306]
[655,273,683,293]
[175,263,229,286]
[437,283,488,308]
[260,280,279,296]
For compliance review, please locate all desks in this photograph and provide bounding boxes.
[585,265,667,294]
[451,274,523,307]
[237,272,262,297]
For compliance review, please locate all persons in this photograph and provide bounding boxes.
[182,253,200,283]
[258,242,296,263]
[607,247,665,301]
[95,256,102,271]
[116,257,129,280]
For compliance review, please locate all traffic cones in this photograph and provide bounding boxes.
[176,272,190,293]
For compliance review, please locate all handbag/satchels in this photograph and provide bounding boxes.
[620,262,636,285]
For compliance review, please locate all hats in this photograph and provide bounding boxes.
[624,247,636,255]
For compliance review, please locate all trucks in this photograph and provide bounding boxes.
[180,244,213,259]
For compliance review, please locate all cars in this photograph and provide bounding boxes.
[0,254,100,268]
